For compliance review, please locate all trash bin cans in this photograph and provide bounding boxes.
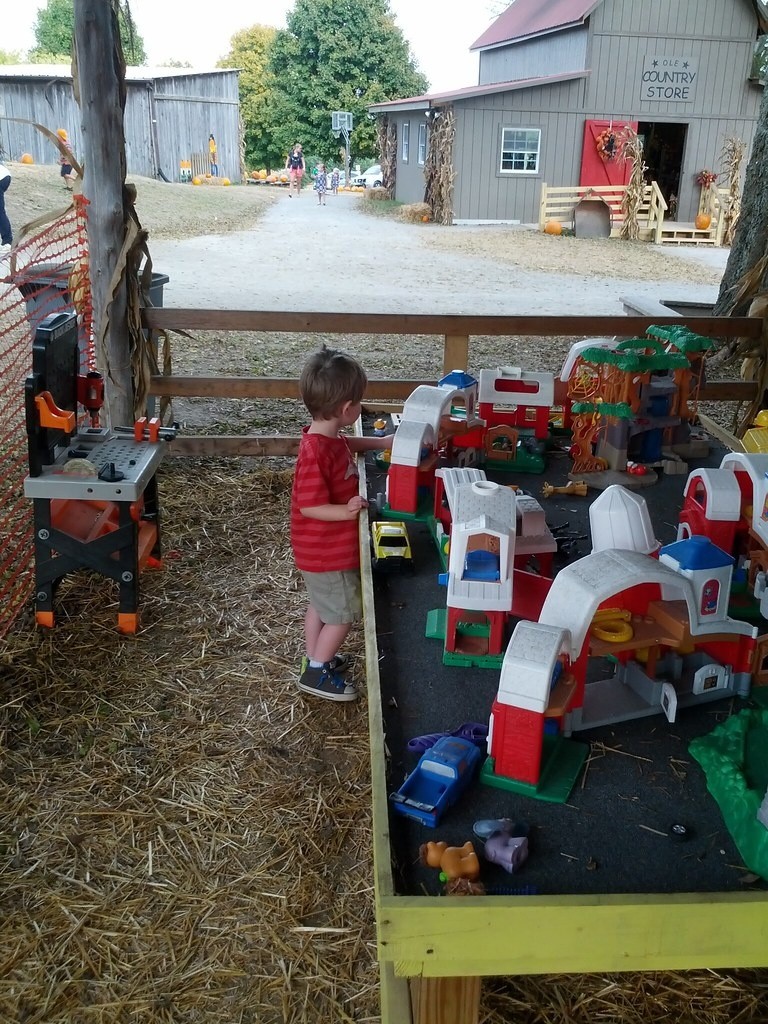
[15,263,168,419]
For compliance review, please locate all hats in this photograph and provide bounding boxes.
[58,128,68,142]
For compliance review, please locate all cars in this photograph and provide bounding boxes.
[350,165,384,189]
[325,171,357,190]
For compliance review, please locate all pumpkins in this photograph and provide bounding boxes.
[252,170,288,183]
[192,177,201,185]
[423,215,429,222]
[337,185,365,192]
[223,178,230,185]
[545,220,562,234]
[21,153,33,164]
[205,173,211,178]
[696,213,710,230]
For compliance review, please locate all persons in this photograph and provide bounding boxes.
[314,164,327,206]
[56,129,75,191]
[330,166,340,195]
[290,345,394,701]
[373,418,386,437]
[286,143,305,197]
[0,165,12,253]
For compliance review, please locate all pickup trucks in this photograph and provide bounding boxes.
[390,736,482,830]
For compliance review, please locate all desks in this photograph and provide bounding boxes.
[356,402,768,1024]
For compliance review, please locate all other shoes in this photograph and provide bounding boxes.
[289,194,293,198]
[300,654,349,674]
[317,202,326,206]
[297,660,358,702]
[0,243,11,253]
[63,187,73,191]
